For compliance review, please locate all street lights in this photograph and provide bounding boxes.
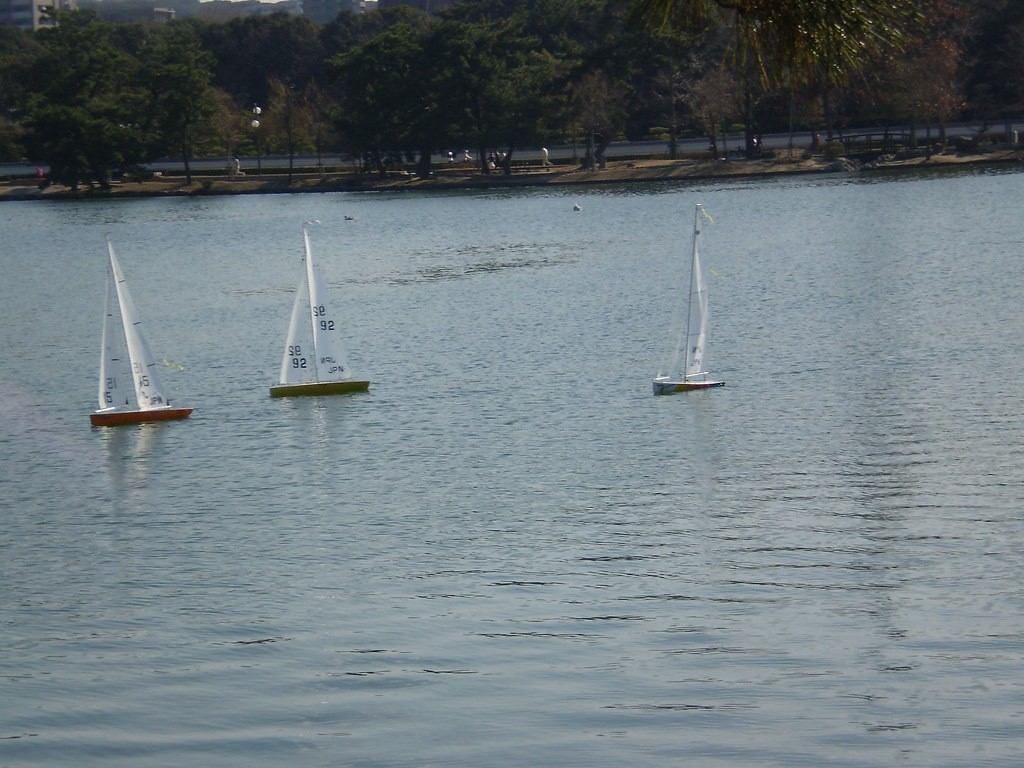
[251,102,263,175]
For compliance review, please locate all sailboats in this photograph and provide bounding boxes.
[652,205,726,398]
[269,222,370,398]
[89,232,194,427]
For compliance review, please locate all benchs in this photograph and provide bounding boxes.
[490,166,556,172]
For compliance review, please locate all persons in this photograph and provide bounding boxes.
[487,150,507,168]
[752,133,763,148]
[464,150,471,163]
[448,150,455,168]
[232,154,246,175]
[541,144,553,166]
[36,165,45,178]
